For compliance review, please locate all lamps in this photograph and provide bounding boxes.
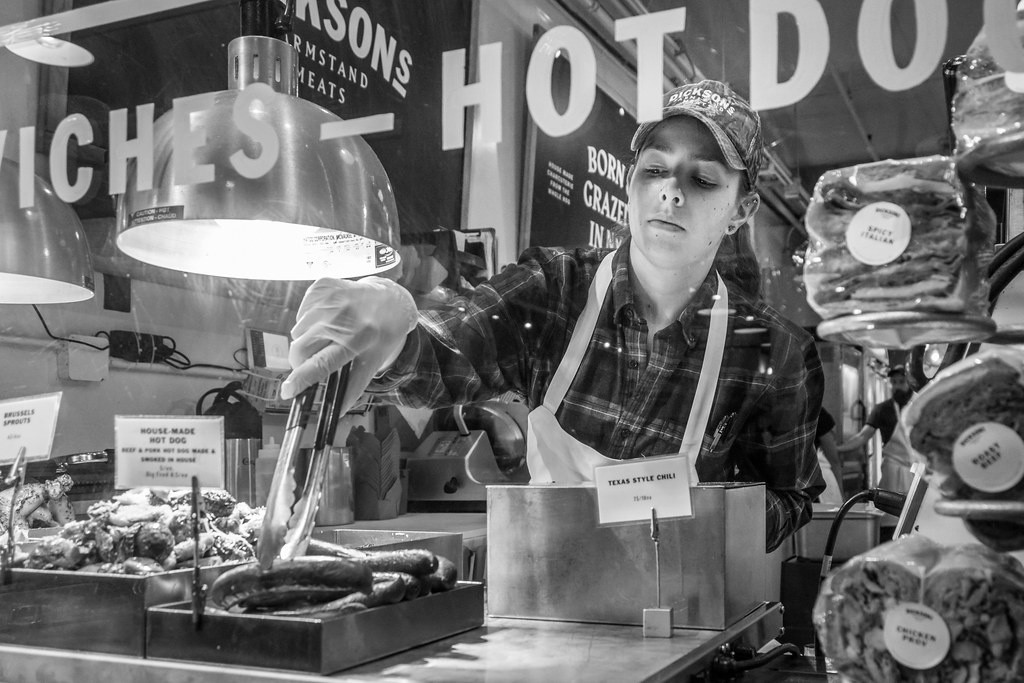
[0,1,402,305]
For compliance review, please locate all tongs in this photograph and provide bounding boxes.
[256,361,352,570]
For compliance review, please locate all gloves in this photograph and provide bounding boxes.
[280,276,420,419]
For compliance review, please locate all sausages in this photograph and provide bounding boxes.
[212,533,459,617]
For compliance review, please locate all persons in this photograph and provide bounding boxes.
[814,407,845,500]
[838,364,926,546]
[281,80,825,557]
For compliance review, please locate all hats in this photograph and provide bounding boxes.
[630,80,764,192]
